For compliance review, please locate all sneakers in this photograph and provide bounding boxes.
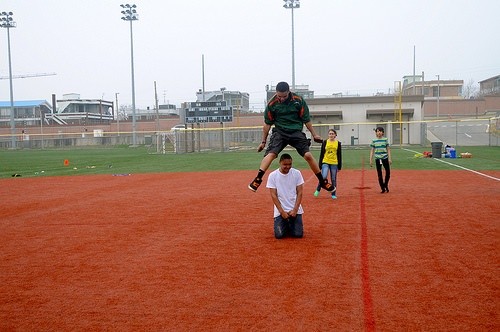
[331,195,337,199]
[249,177,262,191]
[314,190,319,197]
[320,180,336,192]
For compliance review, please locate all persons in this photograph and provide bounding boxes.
[266,153,305,238]
[314,129,342,199]
[248,82,336,192]
[369,127,392,192]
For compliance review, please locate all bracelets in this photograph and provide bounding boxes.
[262,142,266,143]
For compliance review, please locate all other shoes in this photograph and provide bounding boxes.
[381,189,385,193]
[385,184,389,192]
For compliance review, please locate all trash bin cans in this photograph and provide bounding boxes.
[431,142,443,158]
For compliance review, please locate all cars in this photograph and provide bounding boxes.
[171,125,192,130]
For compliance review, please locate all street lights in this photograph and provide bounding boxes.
[0,11,16,148]
[282,0,300,92]
[120,3,140,145]
